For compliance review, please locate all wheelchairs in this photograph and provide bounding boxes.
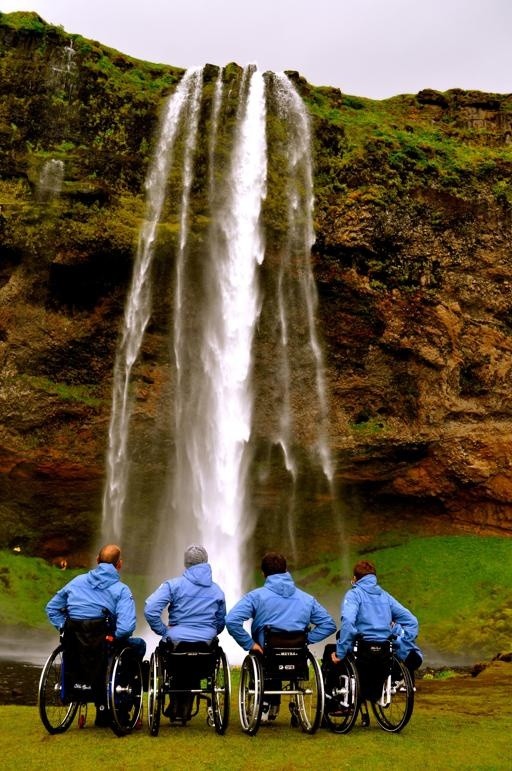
[316,623,419,736]
[35,607,143,740]
[143,637,236,738]
[234,621,325,740]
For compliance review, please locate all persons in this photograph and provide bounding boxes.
[43,541,146,731]
[141,543,226,723]
[319,557,421,717]
[222,549,338,722]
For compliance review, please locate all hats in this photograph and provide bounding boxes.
[183,544,208,565]
